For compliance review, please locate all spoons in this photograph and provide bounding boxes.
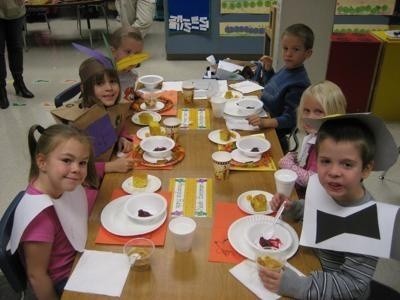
[262,200,287,240]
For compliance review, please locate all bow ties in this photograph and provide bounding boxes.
[317,203,380,243]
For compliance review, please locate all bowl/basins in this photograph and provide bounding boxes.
[124,192,168,224]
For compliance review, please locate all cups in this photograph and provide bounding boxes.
[124,238,154,274]
[168,216,197,254]
[211,151,234,184]
[256,252,285,273]
[246,221,293,255]
[273,168,298,199]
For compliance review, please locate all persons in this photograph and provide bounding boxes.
[277,80,348,199]
[244,23,315,130]
[0,0,35,109]
[6,123,102,300]
[68,55,140,217]
[258,114,382,300]
[109,24,145,104]
[114,0,158,42]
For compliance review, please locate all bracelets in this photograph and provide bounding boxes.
[261,118,264,128]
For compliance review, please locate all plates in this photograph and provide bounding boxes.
[130,74,273,168]
[236,190,277,215]
[121,174,162,194]
[100,193,167,238]
[227,215,300,264]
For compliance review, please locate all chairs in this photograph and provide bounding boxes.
[75,2,110,48]
[22,4,54,51]
[53,80,81,109]
[0,188,29,297]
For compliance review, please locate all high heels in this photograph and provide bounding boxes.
[13,78,34,98]
[0,88,9,109]
